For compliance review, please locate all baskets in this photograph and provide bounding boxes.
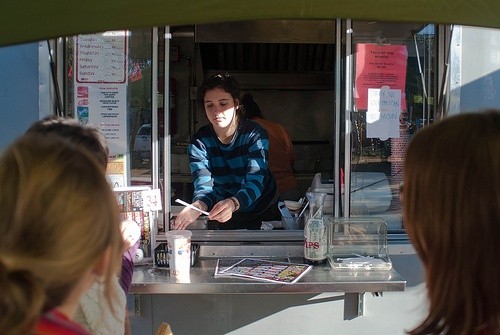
[154,241,200,267]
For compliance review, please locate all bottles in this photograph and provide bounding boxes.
[304,192,329,265]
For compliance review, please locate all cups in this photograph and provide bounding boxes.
[167,231,191,280]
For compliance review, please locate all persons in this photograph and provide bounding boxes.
[175,72,283,231]
[239,94,300,201]
[0,134,124,335]
[402,111,497,335]
[24,115,133,335]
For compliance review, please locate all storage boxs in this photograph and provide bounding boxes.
[328,217,393,270]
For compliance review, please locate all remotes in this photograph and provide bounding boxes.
[278,202,294,223]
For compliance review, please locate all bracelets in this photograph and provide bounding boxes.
[227,196,239,213]
